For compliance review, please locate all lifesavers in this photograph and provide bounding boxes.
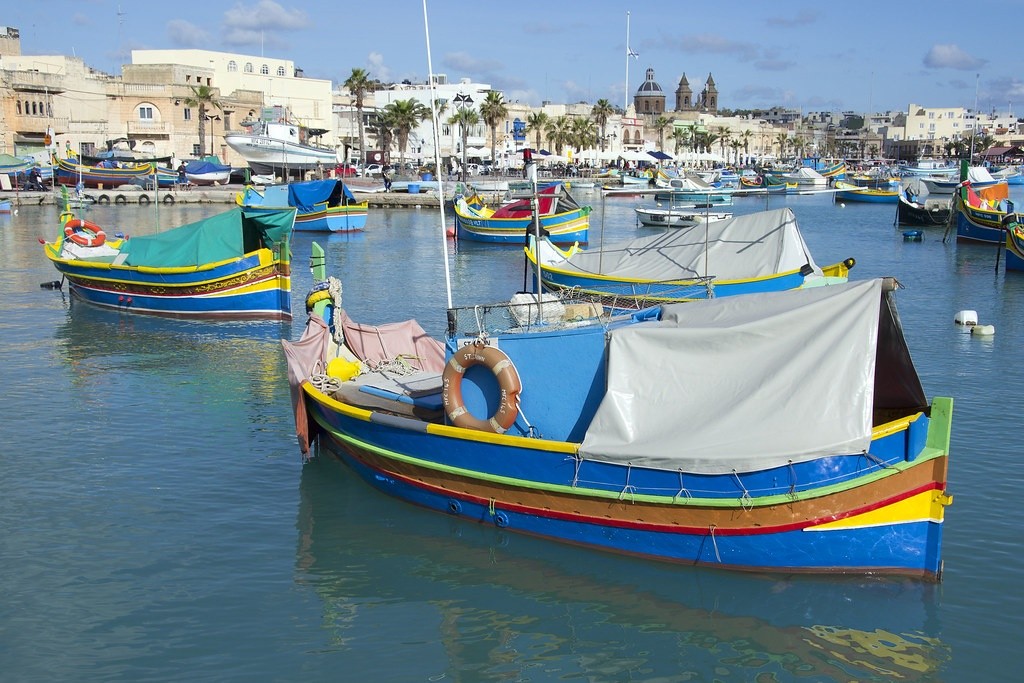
[138,194,150,202]
[163,195,174,202]
[441,344,521,434]
[115,194,126,204]
[755,176,762,185]
[64,219,106,246]
[98,195,110,202]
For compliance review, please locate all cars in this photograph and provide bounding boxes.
[355,162,489,177]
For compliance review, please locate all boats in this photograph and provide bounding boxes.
[897,181,1009,225]
[41,163,299,323]
[155,150,233,186]
[222,29,335,176]
[280,0,954,587]
[955,74,1024,272]
[422,0,856,351]
[633,204,733,227]
[569,128,1024,198]
[0,90,153,189]
[452,106,592,250]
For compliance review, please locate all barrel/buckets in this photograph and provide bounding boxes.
[408,184,419,194]
[422,173,433,181]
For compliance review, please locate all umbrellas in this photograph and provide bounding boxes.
[759,154,776,167]
[390,144,493,168]
[506,147,726,170]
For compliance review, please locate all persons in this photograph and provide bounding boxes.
[519,161,658,177]
[666,161,781,175]
[382,161,393,192]
[944,158,955,165]
[458,164,466,187]
[176,161,188,183]
[18,167,50,191]
[844,162,888,172]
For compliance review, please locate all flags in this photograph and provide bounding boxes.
[628,47,639,59]
